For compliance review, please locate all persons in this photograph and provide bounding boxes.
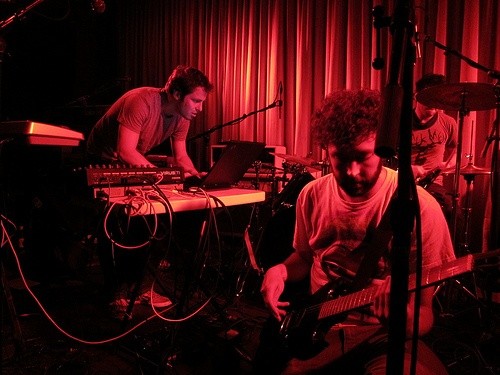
[83,66,209,319]
[259,92,455,374]
[408,73,458,239]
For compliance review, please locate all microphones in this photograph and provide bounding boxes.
[90,0,105,14]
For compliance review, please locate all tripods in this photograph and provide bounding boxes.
[431,195,486,318]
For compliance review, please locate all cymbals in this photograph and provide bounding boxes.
[415,82,496,111]
[439,162,496,178]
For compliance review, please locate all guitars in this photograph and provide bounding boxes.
[261,250,500,359]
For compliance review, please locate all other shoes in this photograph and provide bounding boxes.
[134,291,172,308]
[111,297,132,312]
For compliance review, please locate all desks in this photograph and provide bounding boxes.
[99,189,266,327]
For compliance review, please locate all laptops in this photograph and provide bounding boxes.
[184,140,266,189]
